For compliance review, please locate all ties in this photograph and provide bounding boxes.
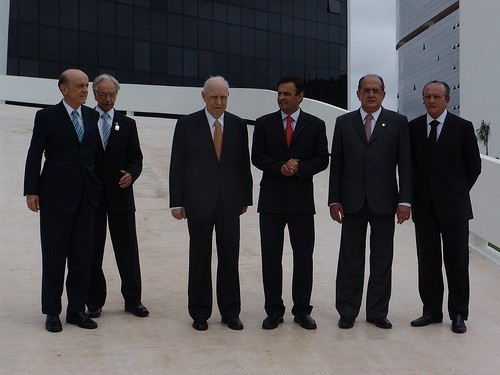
[213,121,223,163]
[284,116,294,147]
[71,110,83,141]
[365,113,372,142]
[101,113,110,150]
[428,120,440,148]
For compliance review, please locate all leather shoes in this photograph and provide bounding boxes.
[262,313,285,330]
[124,303,149,316]
[191,318,209,332]
[367,315,392,329]
[453,313,467,334]
[65,311,96,331]
[337,315,356,329]
[408,311,442,327]
[45,314,62,334]
[293,316,318,331]
[88,308,102,317]
[221,316,245,331]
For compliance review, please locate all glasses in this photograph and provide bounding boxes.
[358,88,383,95]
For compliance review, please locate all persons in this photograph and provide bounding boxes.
[250,75,330,330]
[329,75,411,328]
[168,78,253,332]
[80,72,149,317]
[407,81,482,334]
[22,70,110,332]
